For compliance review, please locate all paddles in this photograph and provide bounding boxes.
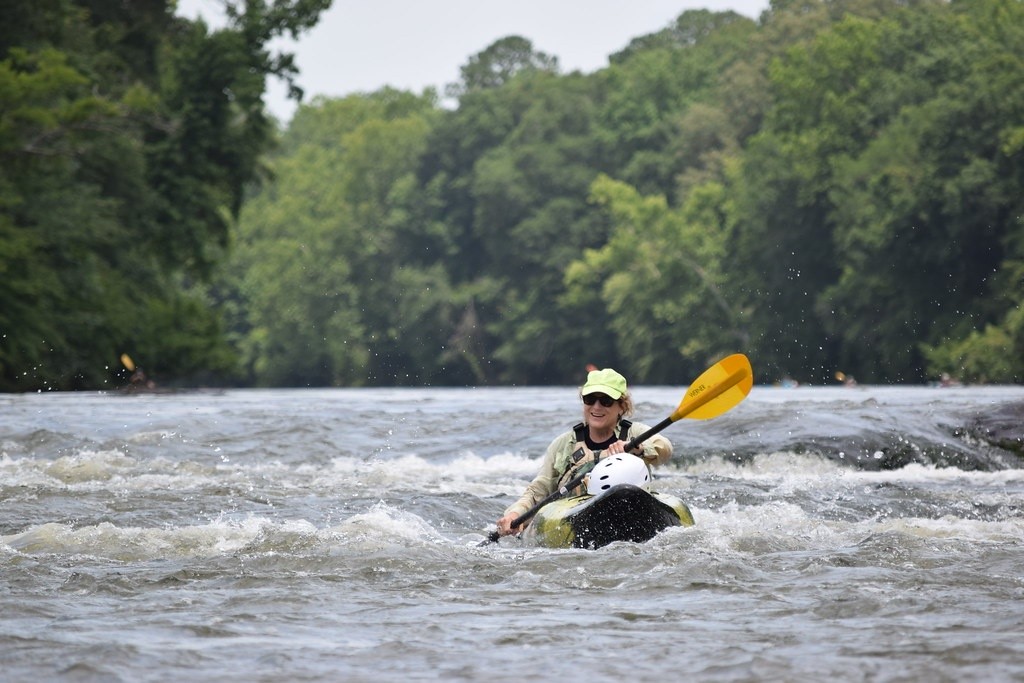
[474,354,754,546]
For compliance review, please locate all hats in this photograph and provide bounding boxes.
[581,368,627,401]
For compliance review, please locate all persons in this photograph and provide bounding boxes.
[496,368,672,536]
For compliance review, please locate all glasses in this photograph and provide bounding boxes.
[583,394,615,407]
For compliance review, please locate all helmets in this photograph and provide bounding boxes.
[587,452,651,496]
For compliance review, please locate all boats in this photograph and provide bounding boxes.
[522,451,696,547]
